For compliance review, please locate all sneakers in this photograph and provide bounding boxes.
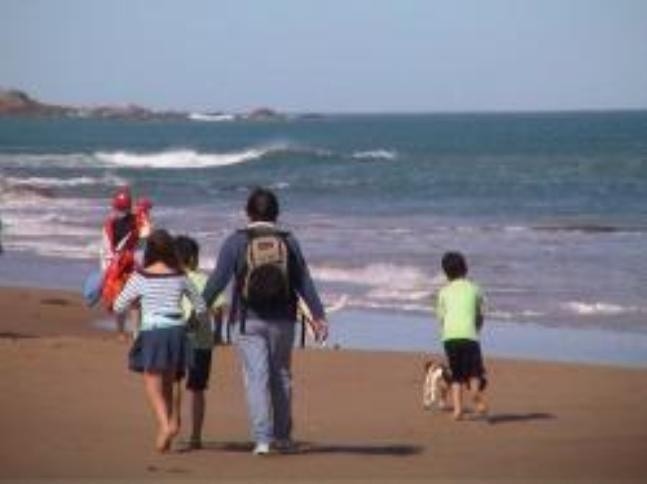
[154,423,202,453]
[254,437,292,455]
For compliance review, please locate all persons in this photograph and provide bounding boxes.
[113,230,213,453]
[203,189,329,455]
[132,199,156,271]
[101,188,142,342]
[174,237,226,447]
[437,251,488,423]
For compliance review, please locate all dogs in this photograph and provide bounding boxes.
[422,356,453,414]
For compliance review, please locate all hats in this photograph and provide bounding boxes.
[110,191,133,208]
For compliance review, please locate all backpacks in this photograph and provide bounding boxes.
[235,229,294,317]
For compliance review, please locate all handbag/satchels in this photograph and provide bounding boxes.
[81,268,107,307]
